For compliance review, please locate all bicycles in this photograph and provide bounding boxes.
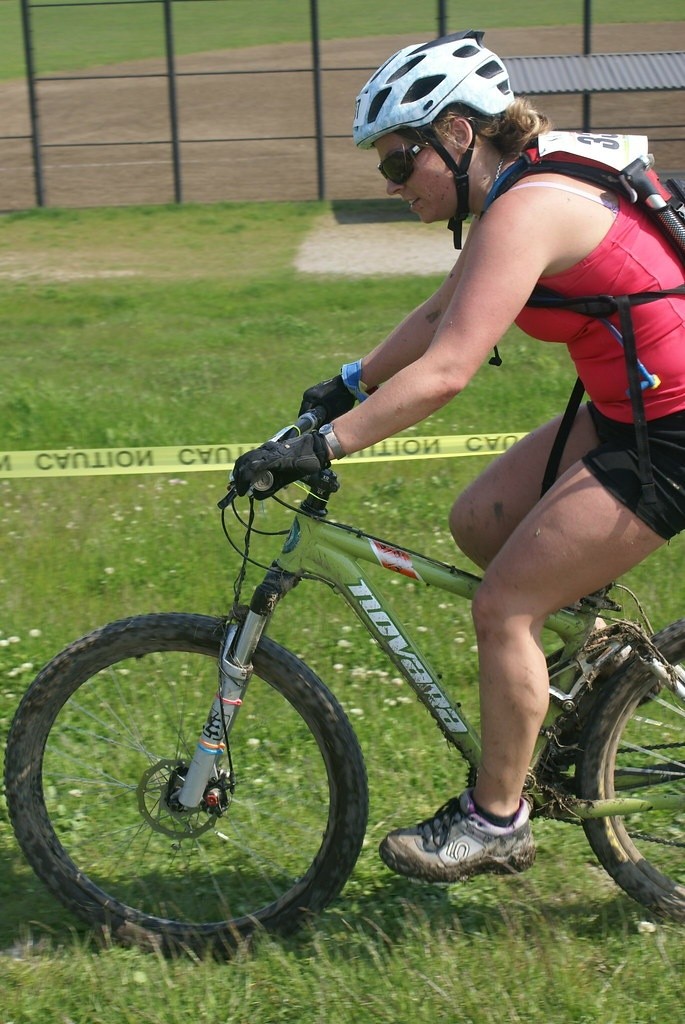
[4,405,685,965]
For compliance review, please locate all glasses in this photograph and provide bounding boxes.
[377,137,429,185]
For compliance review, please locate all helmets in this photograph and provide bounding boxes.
[353,29,516,150]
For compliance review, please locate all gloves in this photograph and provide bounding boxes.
[233,429,330,501]
[298,375,357,430]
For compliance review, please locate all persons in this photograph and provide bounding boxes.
[232,31,685,882]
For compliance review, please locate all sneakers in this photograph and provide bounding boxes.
[545,646,663,707]
[379,788,536,886]
